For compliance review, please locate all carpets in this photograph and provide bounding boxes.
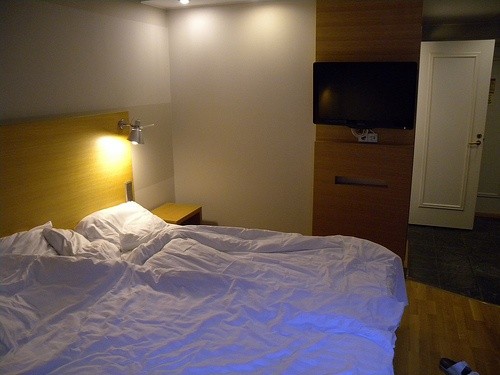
[407,216,500,306]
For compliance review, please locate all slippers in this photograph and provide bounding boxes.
[440,358,479,375]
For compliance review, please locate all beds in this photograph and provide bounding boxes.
[0,111,408,375]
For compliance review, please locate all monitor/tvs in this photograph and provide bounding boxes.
[311,62,419,131]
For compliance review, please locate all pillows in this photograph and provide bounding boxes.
[0,220,59,256]
[75,200,167,253]
[43,228,89,256]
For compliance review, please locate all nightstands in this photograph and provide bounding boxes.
[150,201,202,225]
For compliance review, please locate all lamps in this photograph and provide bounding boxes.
[118,118,141,143]
